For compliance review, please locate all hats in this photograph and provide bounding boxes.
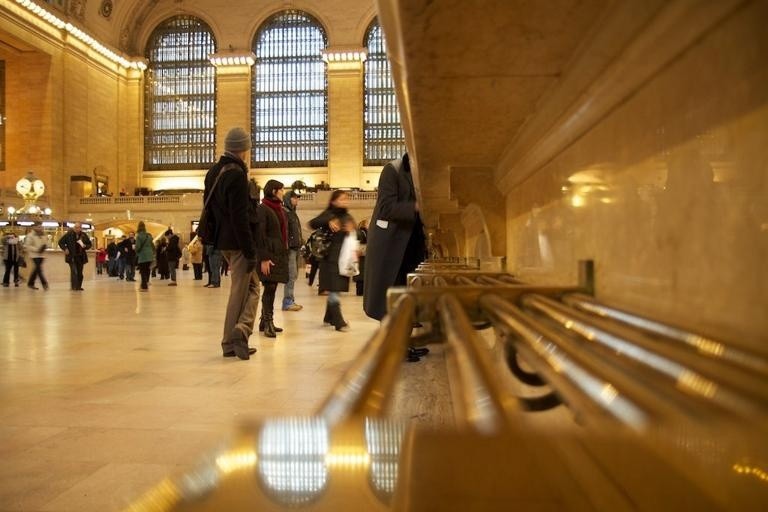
[290,191,302,199]
[223,126,250,152]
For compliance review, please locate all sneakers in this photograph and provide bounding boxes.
[28,285,39,290]
[203,282,220,288]
[43,282,48,289]
[96,270,178,292]
[282,304,303,312]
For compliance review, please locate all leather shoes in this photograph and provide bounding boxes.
[259,318,284,338]
[222,330,257,360]
[407,347,430,363]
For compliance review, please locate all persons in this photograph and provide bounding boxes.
[96,220,229,291]
[25,222,51,290]
[1,234,23,286]
[306,219,368,296]
[363,153,429,363]
[305,190,358,331]
[197,127,303,362]
[58,222,92,290]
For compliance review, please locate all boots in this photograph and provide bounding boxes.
[323,300,349,331]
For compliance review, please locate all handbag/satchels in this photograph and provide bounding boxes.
[197,207,217,245]
[311,227,332,258]
[17,256,27,267]
[358,243,367,257]
